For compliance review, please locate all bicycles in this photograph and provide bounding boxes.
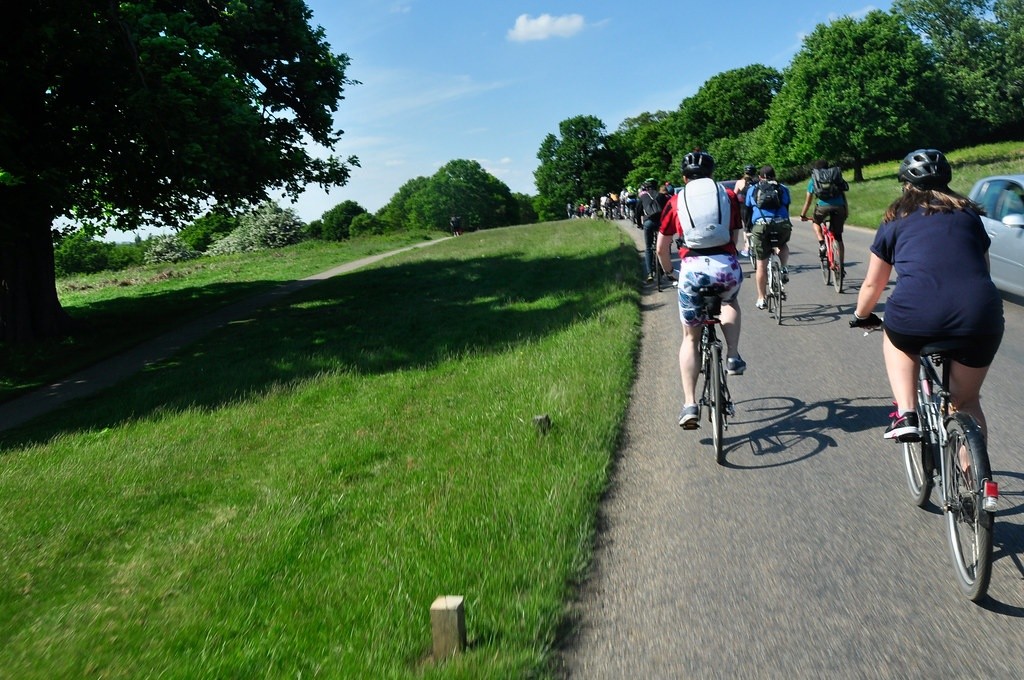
[802,217,846,293]
[699,287,735,463]
[637,225,664,292]
[849,320,999,602]
[748,231,789,325]
[569,205,644,228]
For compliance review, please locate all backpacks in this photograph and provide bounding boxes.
[643,191,662,218]
[811,166,844,200]
[678,177,730,248]
[755,181,783,211]
[741,177,757,196]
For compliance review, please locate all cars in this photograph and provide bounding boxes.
[968,174,1024,297]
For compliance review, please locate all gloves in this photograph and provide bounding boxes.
[852,312,882,328]
[665,268,679,281]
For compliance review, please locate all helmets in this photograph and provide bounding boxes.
[745,166,757,175]
[898,148,952,189]
[681,152,714,176]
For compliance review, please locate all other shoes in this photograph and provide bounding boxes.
[780,267,791,282]
[741,251,748,257]
[755,299,768,309]
[646,276,653,283]
[818,245,827,256]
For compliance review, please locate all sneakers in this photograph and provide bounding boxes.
[726,352,747,375]
[883,403,920,437]
[679,403,700,425]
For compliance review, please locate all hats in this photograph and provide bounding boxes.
[758,166,776,180]
[814,160,829,171]
[645,178,659,189]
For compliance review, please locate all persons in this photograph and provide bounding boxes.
[800,160,847,262]
[665,181,674,194]
[744,165,793,309]
[735,165,759,257]
[854,149,1005,486]
[656,152,747,426]
[600,188,644,228]
[635,178,668,282]
[578,200,596,217]
[660,185,674,199]
[450,216,460,237]
